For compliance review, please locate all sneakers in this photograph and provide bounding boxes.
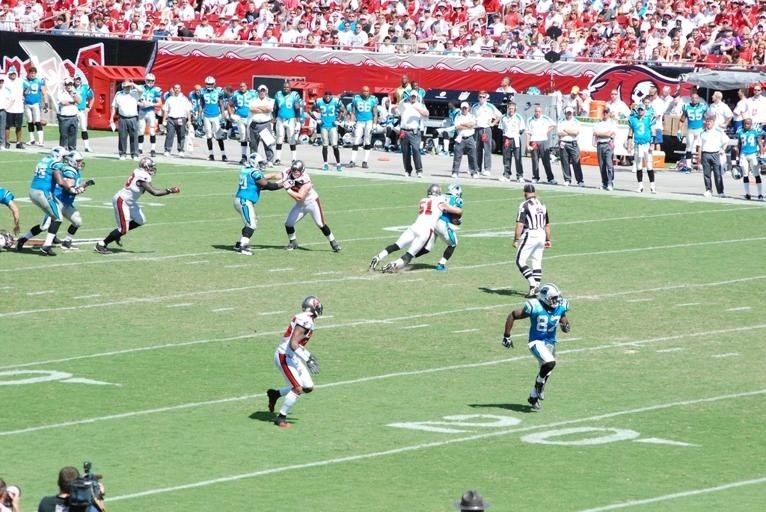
[451,173,458,178]
[94,243,113,254]
[380,262,396,274]
[367,257,380,274]
[434,263,449,272]
[469,168,491,179]
[114,237,123,247]
[15,239,23,252]
[267,388,280,412]
[274,417,295,429]
[528,397,543,411]
[535,377,547,400]
[330,239,342,251]
[284,241,298,251]
[525,287,540,298]
[39,234,79,256]
[403,170,423,178]
[233,240,254,255]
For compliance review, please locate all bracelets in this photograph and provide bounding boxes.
[545,240,550,241]
[302,348,309,357]
[293,348,309,364]
[513,238,519,242]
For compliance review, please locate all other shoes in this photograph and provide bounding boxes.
[599,184,616,192]
[4,138,44,149]
[116,148,185,161]
[500,174,586,187]
[704,189,764,201]
[635,185,657,194]
[207,152,282,168]
[322,159,368,172]
[84,145,90,152]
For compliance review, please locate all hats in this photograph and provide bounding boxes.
[0,0,766,57]
[74,73,82,79]
[563,85,698,114]
[64,78,74,84]
[401,75,409,84]
[461,89,543,114]
[8,67,17,74]
[410,90,418,98]
[452,489,491,511]
[523,184,535,193]
[325,91,332,95]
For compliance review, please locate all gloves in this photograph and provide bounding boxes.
[74,186,85,195]
[84,179,95,187]
[307,354,320,375]
[502,335,514,349]
[167,186,180,194]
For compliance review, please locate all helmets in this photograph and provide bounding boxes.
[302,296,323,318]
[50,145,69,161]
[145,73,155,87]
[448,183,462,197]
[291,160,304,172]
[427,184,441,196]
[248,153,266,171]
[139,156,156,176]
[258,85,269,94]
[539,283,563,308]
[122,80,133,90]
[64,149,84,168]
[205,76,216,90]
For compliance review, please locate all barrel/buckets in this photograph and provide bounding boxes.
[145,119,160,135]
[589,100,605,119]
[653,150,666,169]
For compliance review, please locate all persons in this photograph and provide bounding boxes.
[278,159,343,253]
[15,151,96,251]
[93,155,181,255]
[511,181,551,298]
[28,145,73,256]
[36,465,107,512]
[0,188,21,251]
[367,182,464,275]
[0,0,766,71]
[231,151,286,256]
[501,282,570,413]
[0,66,428,177]
[0,478,22,512]
[406,182,463,272]
[451,488,493,512]
[265,296,324,430]
[432,77,766,200]
